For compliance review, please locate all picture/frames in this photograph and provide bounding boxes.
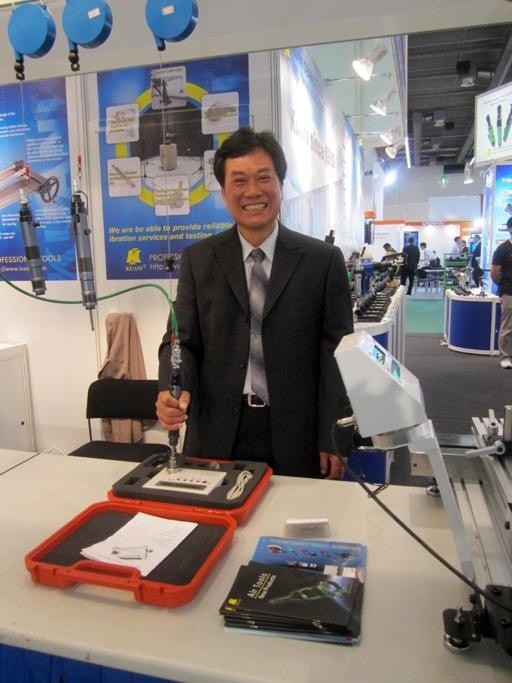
[473,80,512,168]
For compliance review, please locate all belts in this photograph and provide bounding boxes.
[241,393,268,409]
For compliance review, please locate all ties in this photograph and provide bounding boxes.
[249,249,270,406]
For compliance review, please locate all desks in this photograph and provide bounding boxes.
[443,288,502,357]
[351,283,407,396]
[425,269,443,295]
[0,448,39,475]
[0,342,36,452]
[0,454,511,683]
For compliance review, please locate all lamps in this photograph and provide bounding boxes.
[463,162,474,184]
[351,34,409,159]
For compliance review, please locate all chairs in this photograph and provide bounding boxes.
[67,378,172,464]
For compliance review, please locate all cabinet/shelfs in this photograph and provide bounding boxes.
[443,252,467,297]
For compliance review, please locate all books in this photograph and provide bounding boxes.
[220,535,367,645]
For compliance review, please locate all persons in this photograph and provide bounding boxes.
[382,232,481,295]
[490,215,511,372]
[148,123,353,480]
